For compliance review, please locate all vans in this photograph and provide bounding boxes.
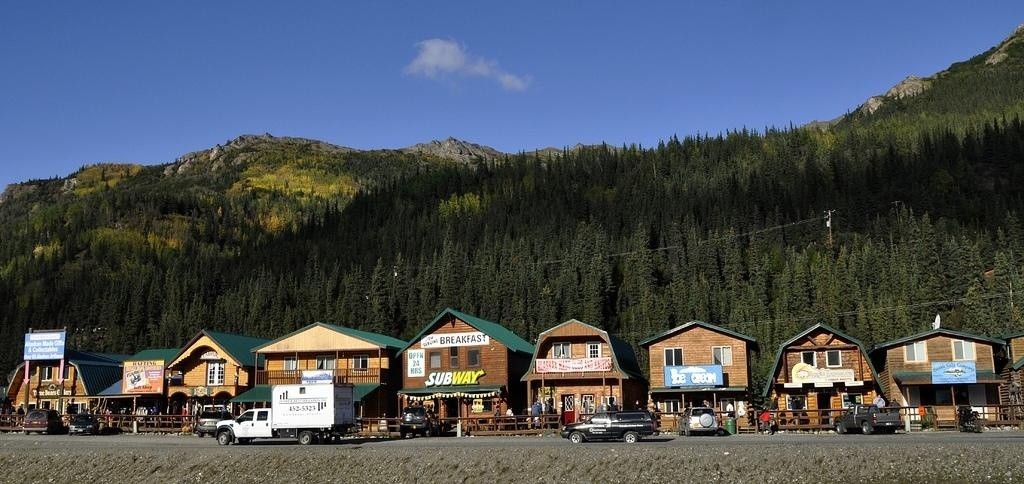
[23,408,64,435]
[198,410,234,438]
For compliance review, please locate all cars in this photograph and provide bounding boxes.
[68,412,100,436]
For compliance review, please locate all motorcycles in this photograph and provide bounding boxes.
[955,406,984,434]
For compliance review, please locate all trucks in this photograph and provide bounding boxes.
[832,402,904,436]
[213,380,357,446]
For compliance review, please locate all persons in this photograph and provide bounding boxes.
[493,397,553,430]
[888,398,902,406]
[759,408,772,435]
[0,397,248,426]
[873,393,885,407]
[725,399,733,411]
[767,419,778,435]
[407,399,438,421]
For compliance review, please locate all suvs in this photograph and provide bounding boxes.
[399,404,442,437]
[557,407,660,444]
[677,405,719,436]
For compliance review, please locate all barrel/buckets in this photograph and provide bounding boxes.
[725,420,735,435]
